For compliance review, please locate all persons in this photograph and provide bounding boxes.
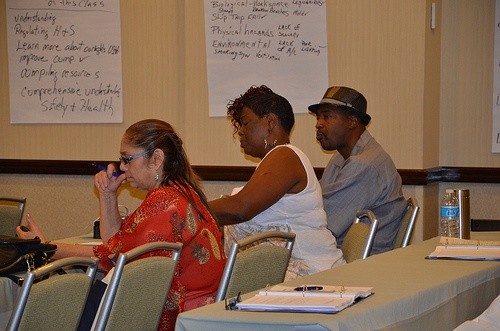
[208,85,348,275]
[308,86,406,257]
[16,118,227,331]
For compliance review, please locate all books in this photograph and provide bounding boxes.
[237,284,375,314]
[426,236,500,261]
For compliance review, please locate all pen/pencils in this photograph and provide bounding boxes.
[294,286,324,291]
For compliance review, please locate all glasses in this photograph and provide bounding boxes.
[118,150,151,163]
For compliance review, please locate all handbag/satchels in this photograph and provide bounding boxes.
[0,234,66,287]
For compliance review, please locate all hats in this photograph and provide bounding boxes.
[308,86,371,126]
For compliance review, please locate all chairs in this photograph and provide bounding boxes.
[341,209,380,264]
[393,197,420,250]
[214,232,297,302]
[0,196,27,237]
[94,241,183,331]
[5,256,99,331]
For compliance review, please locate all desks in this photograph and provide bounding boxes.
[0,233,103,331]
[175,229,500,331]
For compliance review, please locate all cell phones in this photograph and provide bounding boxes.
[20,225,30,232]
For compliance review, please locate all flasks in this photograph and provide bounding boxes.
[457,189,471,240]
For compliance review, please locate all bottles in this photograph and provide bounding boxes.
[440,189,460,239]
